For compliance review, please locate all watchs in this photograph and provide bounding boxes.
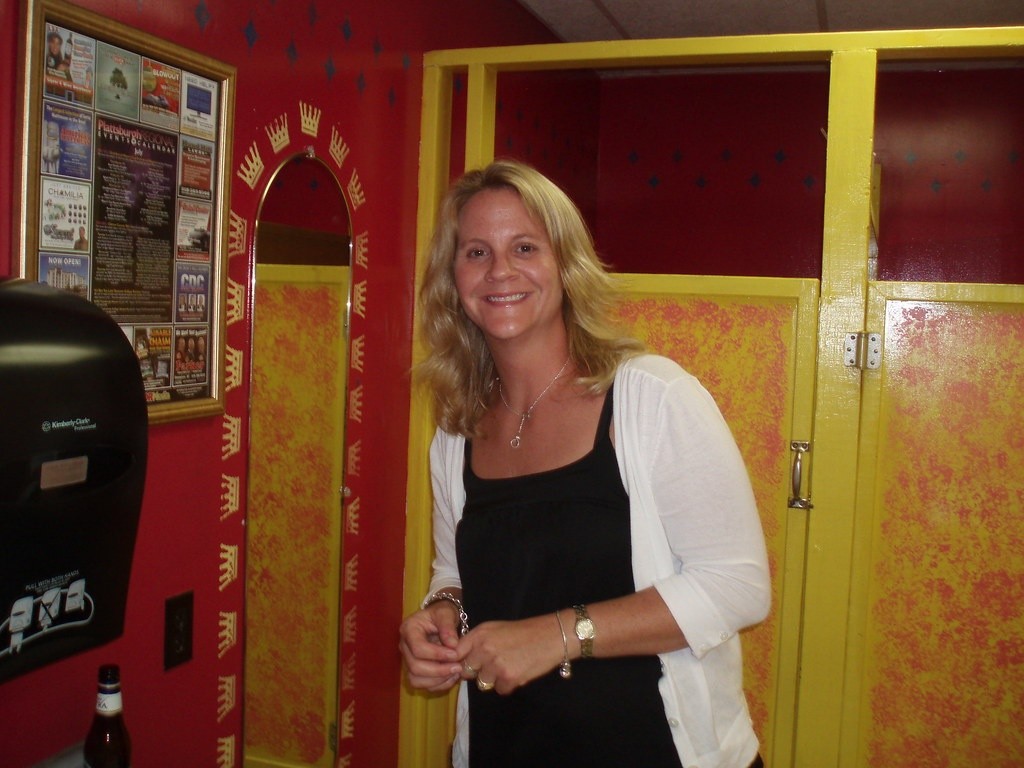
[573,604,595,658]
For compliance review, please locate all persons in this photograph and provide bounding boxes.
[177,336,206,361]
[74,227,88,251]
[44,199,53,214]
[48,32,73,82]
[179,295,204,311]
[398,162,770,768]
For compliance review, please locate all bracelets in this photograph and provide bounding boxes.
[420,593,469,637]
[556,611,571,677]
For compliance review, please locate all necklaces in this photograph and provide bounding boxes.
[499,356,570,448]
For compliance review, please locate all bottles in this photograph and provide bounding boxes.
[83,665,132,768]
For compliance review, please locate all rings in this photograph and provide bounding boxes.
[477,675,495,690]
[464,659,479,673]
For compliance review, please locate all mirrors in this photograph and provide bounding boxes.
[240,149,355,768]
